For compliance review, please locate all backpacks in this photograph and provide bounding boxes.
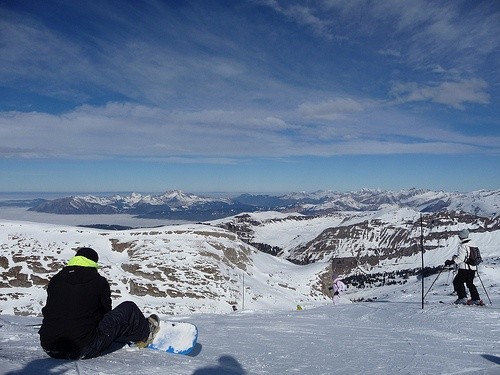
[464,246,482,266]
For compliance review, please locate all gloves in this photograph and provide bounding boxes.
[445,259,455,267]
[452,254,457,260]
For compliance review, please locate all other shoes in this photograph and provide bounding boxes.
[453,297,468,305]
[467,299,481,306]
[135,314,160,348]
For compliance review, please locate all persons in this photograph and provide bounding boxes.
[39,247,159,362]
[445,229,480,305]
[297,305,302,310]
[328,274,342,305]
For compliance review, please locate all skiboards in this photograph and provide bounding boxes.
[439,301,485,306]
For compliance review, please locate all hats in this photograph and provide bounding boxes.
[459,229,469,239]
[74,247,99,263]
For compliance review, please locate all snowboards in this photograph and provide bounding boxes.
[146,320,198,355]
